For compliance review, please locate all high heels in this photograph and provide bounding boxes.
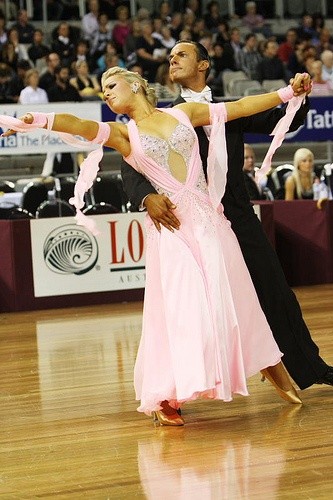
[154,410,184,427]
[260,369,302,404]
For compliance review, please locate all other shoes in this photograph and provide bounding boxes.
[315,366,333,386]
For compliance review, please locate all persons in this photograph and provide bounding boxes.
[41,152,81,179]
[120,39,333,390]
[242,142,276,200]
[0,0,333,111]
[1,66,312,427]
[284,148,319,202]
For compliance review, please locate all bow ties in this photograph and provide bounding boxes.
[191,91,213,103]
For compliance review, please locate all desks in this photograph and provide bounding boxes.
[0,96,333,156]
[0,200,333,313]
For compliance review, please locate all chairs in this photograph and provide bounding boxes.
[0,163,333,220]
[222,71,285,97]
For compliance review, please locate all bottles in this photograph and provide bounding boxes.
[127,201,132,213]
[313,183,320,201]
[319,180,328,200]
[254,168,267,183]
[263,188,270,201]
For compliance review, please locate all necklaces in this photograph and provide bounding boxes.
[135,109,164,130]
